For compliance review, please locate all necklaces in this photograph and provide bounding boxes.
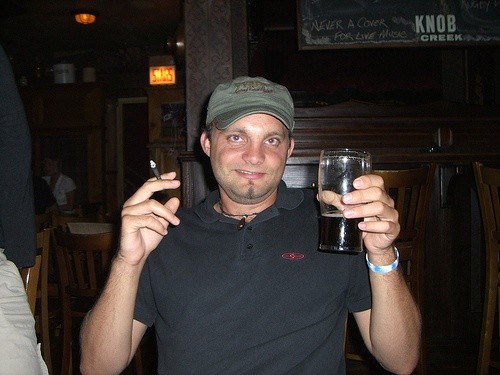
[219,201,259,231]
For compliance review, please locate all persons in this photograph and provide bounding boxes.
[41,156,77,211]
[31,161,58,214]
[81,75,423,375]
[0,247,43,375]
[0,47,36,266]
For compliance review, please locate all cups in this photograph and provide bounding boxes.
[83,67,96,82]
[53,63,74,84]
[318,148,372,252]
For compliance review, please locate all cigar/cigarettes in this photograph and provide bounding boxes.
[150,160,162,180]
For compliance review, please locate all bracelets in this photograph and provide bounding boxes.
[366,245,399,274]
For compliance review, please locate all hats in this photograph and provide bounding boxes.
[205,76,295,133]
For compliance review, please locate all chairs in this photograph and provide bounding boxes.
[475,162,500,375]
[373,166,432,286]
[51,216,141,375]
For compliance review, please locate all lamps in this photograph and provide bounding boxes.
[148,55,177,87]
[74,5,96,26]
